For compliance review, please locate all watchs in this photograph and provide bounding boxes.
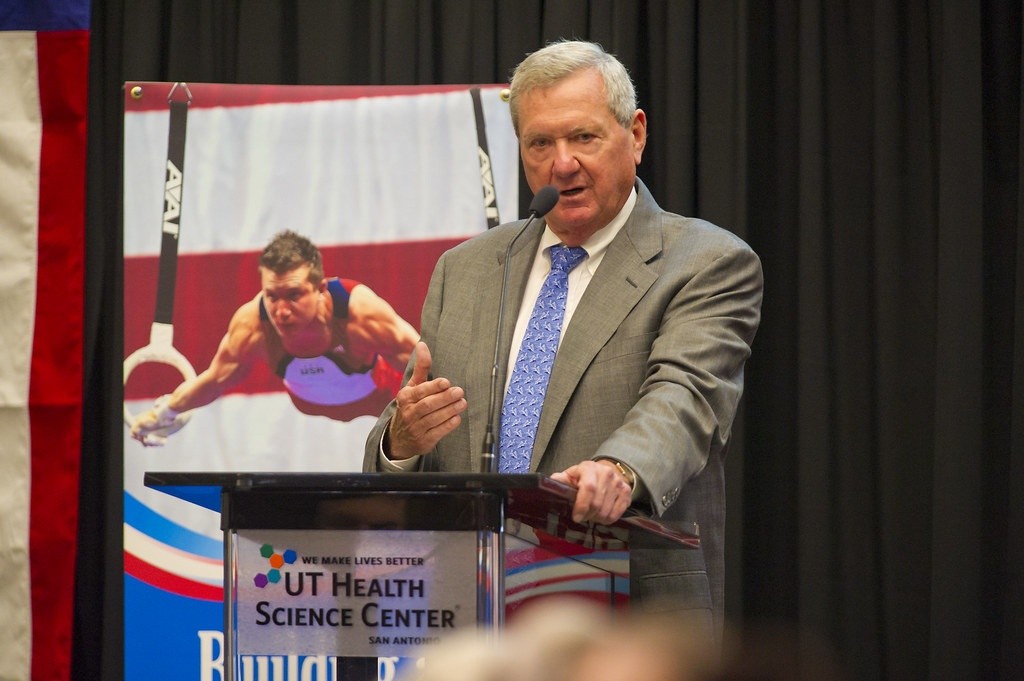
[595,456,634,490]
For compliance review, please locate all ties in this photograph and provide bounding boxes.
[495,244,589,471]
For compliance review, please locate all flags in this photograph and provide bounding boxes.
[0,1,97,681]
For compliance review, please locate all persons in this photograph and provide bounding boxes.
[129,223,421,448]
[359,39,766,636]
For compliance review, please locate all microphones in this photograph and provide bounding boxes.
[479,184,559,475]
